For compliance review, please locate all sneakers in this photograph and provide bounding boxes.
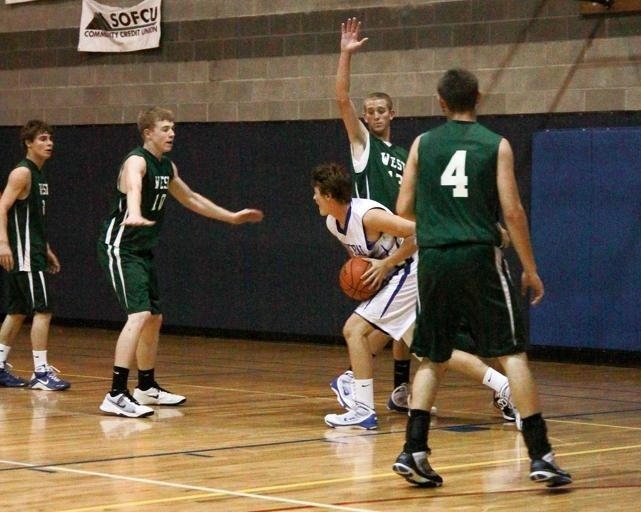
[501,381,523,431]
[0,368,29,386]
[330,373,357,410]
[492,393,515,421]
[531,446,573,487]
[133,385,187,405]
[325,402,378,430]
[99,390,154,418]
[387,388,437,414]
[30,369,71,390]
[391,447,443,486]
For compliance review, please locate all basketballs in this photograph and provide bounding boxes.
[339,255,380,300]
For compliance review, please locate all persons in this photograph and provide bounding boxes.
[393,69,574,487]
[96,106,264,418]
[329,17,438,414]
[312,163,516,429]
[1,120,72,390]
